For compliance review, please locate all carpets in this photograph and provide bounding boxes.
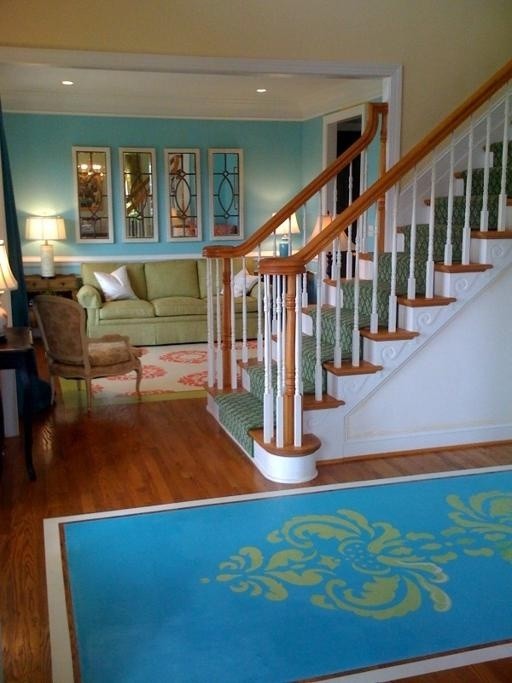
[57,336,264,406]
[41,464,512,683]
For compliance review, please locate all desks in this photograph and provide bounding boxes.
[1,325,38,485]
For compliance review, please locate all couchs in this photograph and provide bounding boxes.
[77,254,280,346]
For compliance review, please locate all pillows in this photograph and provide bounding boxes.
[220,267,261,298]
[93,264,140,303]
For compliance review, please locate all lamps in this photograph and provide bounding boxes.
[0,239,19,348]
[269,212,300,258]
[24,213,68,281]
[308,217,357,280]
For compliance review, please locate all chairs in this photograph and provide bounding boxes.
[29,294,145,413]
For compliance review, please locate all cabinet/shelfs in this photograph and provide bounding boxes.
[22,275,78,338]
